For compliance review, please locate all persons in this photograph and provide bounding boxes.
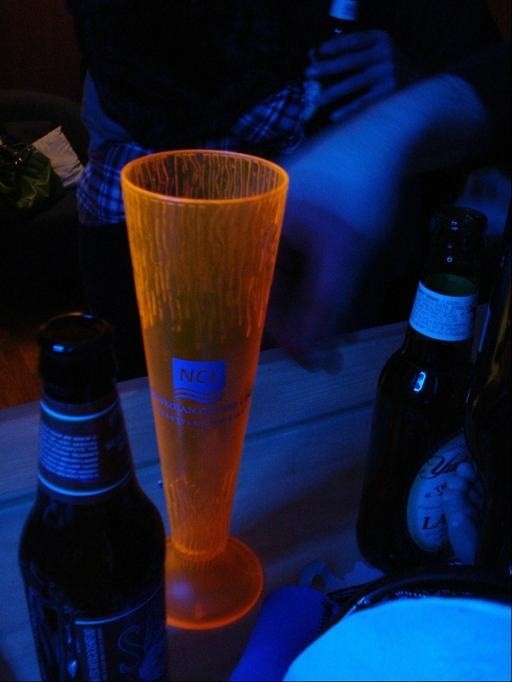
[1,2,512,372]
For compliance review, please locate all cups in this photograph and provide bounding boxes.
[118,146,293,633]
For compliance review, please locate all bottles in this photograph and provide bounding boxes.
[324,0,358,44]
[353,202,490,580]
[18,313,169,679]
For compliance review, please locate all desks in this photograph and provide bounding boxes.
[0,302,488,682]
